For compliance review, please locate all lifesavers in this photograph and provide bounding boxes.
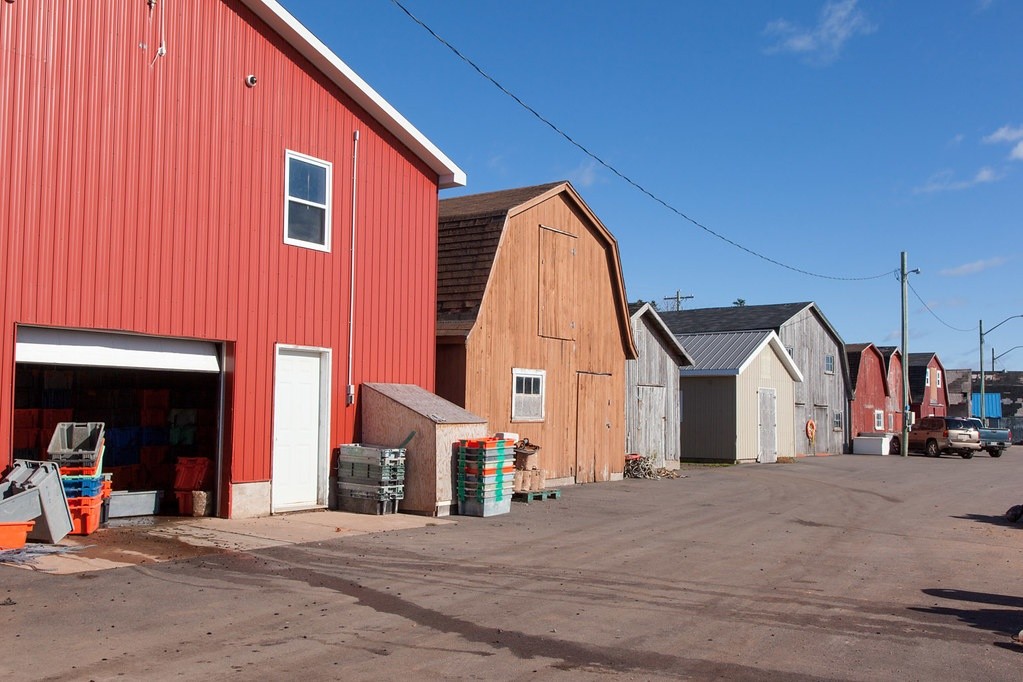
[807,420,816,438]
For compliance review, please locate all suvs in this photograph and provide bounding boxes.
[891,416,983,460]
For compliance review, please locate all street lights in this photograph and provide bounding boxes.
[901,267,921,455]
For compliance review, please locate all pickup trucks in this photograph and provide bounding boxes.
[968,417,1012,458]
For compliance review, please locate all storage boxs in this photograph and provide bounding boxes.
[337,442,407,515]
[0,421,213,551]
[456,438,517,517]
